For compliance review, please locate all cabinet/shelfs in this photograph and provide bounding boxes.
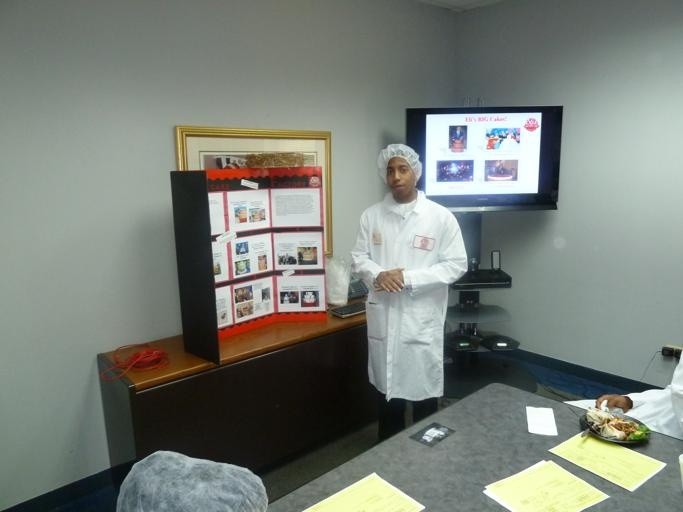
[443,204,556,398]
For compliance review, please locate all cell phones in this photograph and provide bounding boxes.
[330,301,364,318]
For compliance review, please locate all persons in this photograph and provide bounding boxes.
[451,126,464,143]
[487,130,520,149]
[349,144,468,446]
[563,353,683,439]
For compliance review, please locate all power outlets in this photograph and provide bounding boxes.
[662,345,682,359]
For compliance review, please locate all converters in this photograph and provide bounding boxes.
[661,347,674,356]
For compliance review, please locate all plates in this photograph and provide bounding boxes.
[579,412,651,444]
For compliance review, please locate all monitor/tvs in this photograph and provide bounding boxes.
[406,106,563,213]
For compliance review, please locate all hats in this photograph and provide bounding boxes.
[377,144,422,184]
[116,451,267,512]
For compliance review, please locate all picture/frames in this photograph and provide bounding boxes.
[176,125,332,258]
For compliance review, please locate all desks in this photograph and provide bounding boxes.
[96,295,378,493]
[267,383,683,512]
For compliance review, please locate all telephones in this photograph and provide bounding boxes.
[347,275,368,300]
